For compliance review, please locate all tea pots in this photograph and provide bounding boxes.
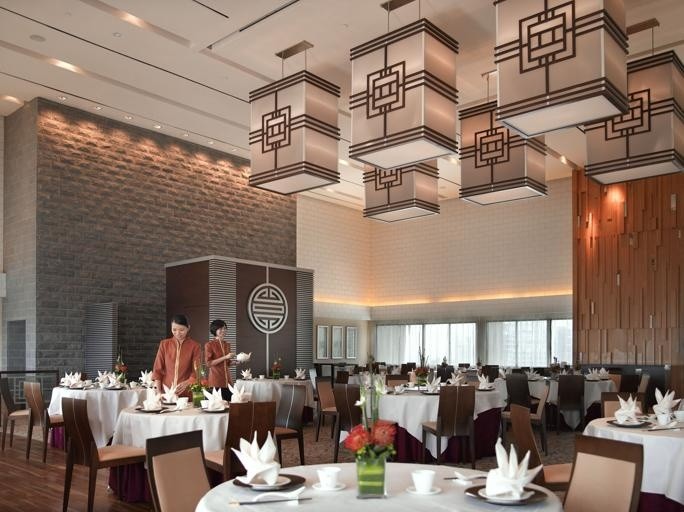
[233,351,252,361]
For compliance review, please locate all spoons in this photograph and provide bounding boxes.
[250,485,306,503]
[454,470,488,479]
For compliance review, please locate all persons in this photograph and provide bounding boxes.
[152,312,203,399]
[204,320,235,388]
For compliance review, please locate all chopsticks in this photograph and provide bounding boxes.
[647,426,684,431]
[443,476,486,480]
[239,496,312,504]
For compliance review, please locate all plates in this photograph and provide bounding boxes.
[232,473,307,492]
[57,381,156,390]
[606,411,653,428]
[422,387,495,395]
[498,376,610,382]
[134,400,232,415]
[464,483,550,507]
[240,377,307,381]
[312,483,346,491]
[406,484,441,495]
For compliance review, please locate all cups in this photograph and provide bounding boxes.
[394,385,405,393]
[410,468,436,492]
[259,374,289,380]
[674,410,683,422]
[316,465,343,490]
[176,397,189,407]
[440,383,446,386]
[83,379,92,385]
[657,412,670,425]
[406,382,415,389]
